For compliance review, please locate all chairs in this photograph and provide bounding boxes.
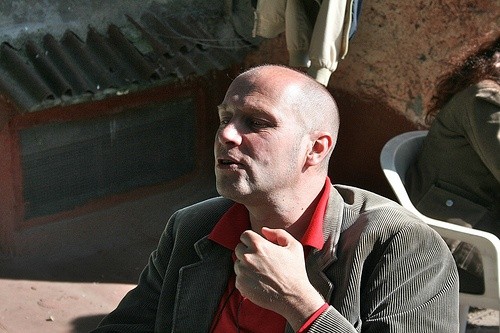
[380,131,500,333]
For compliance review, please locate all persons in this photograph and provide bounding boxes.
[93,64,460,333]
[405,35,500,294]
